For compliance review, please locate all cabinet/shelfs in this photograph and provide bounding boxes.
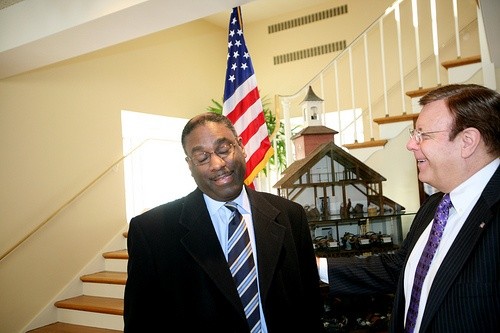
[272,141,406,333]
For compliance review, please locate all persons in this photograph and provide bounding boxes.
[124,112,321,333]
[316,84,500,333]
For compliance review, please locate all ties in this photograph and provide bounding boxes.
[404,193,453,333]
[223,202,262,333]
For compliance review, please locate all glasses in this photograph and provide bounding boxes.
[409,129,462,144]
[187,141,238,166]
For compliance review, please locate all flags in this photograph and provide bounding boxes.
[221,6,275,191]
